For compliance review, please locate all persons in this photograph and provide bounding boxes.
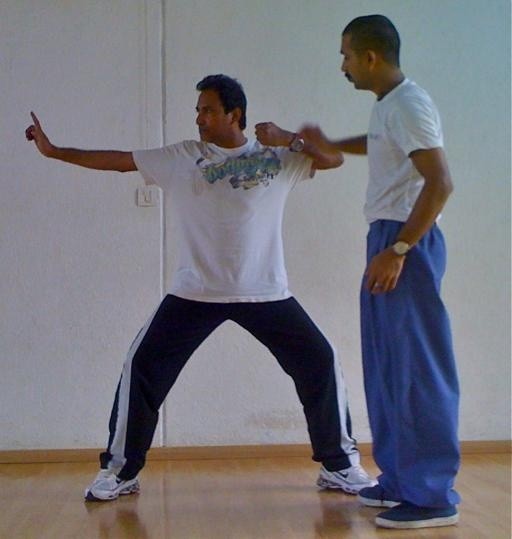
[302,15,460,528]
[25,74,379,501]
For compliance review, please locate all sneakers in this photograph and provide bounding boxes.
[317,463,459,530]
[84,469,140,501]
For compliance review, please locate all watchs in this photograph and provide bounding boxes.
[392,240,410,256]
[291,133,303,153]
